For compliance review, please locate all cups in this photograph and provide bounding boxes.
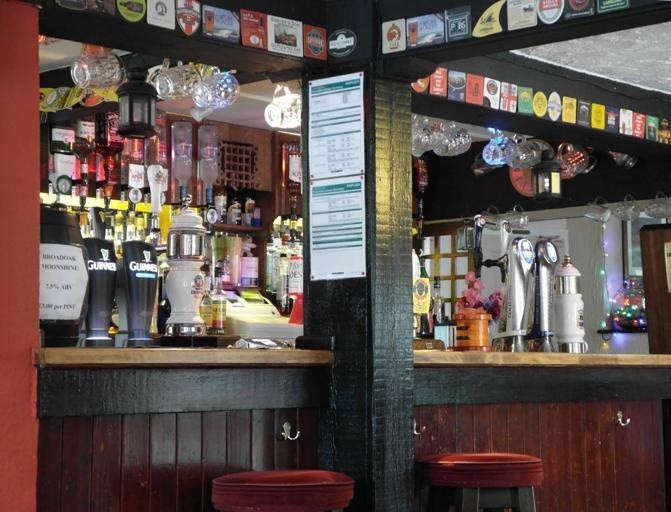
[412,115,472,157]
[80,187,88,196]
[615,195,640,222]
[150,55,202,99]
[241,258,259,288]
[507,205,529,230]
[557,342,588,353]
[71,43,126,88]
[558,141,588,180]
[192,64,240,109]
[243,214,253,225]
[583,197,611,223]
[482,206,503,227]
[71,186,78,196]
[483,127,540,168]
[265,83,303,130]
[645,192,671,220]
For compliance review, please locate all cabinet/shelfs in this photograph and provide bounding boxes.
[213,188,269,293]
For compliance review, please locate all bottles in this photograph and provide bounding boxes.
[96,102,125,181]
[74,179,90,221]
[420,258,430,282]
[119,139,146,191]
[127,201,137,218]
[213,232,230,286]
[207,266,226,335]
[173,206,204,217]
[197,124,219,205]
[228,190,242,224]
[146,125,168,213]
[114,214,125,259]
[202,235,213,258]
[200,275,214,335]
[74,109,96,178]
[283,141,303,210]
[172,120,193,202]
[150,214,161,247]
[97,197,116,224]
[215,186,226,223]
[135,214,145,241]
[413,314,418,338]
[420,313,429,338]
[216,261,225,290]
[49,125,76,154]
[245,197,255,213]
[79,153,88,179]
[90,142,102,183]
[230,234,242,287]
[126,218,135,242]
[430,275,442,325]
[265,212,302,316]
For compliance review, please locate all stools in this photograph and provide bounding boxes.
[421,452,544,512]
[211,470,355,511]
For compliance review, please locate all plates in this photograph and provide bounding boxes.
[80,98,104,107]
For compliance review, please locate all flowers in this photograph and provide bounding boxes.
[456,271,502,323]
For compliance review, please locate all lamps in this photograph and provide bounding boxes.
[531,140,561,199]
[115,54,157,140]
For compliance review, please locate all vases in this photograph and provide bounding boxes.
[452,314,493,349]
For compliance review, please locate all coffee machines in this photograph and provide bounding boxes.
[166,194,207,337]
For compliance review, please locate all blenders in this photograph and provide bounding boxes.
[552,255,586,342]
